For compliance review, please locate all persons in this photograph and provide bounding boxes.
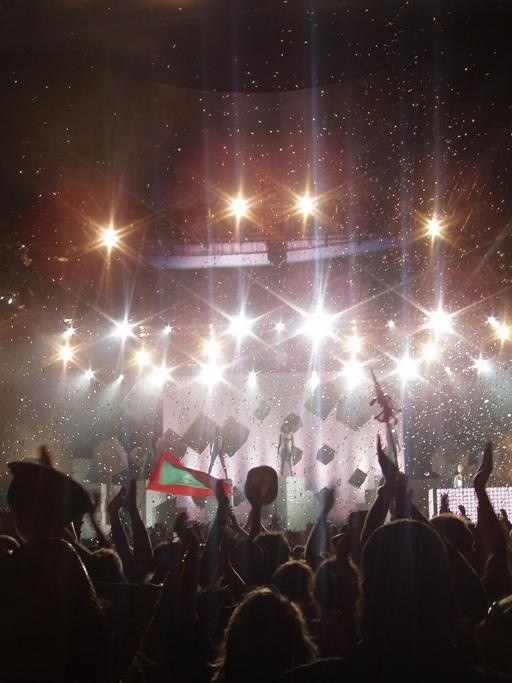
[1,422,511,682]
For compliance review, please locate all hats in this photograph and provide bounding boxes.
[6,462,94,538]
